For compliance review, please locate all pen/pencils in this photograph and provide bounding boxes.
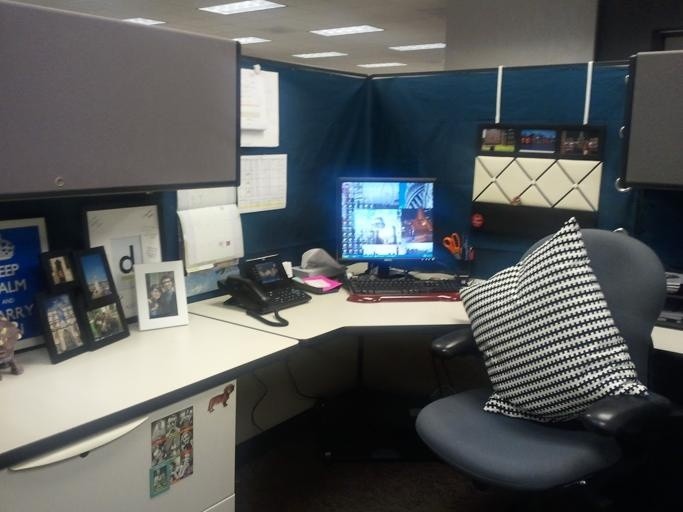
[461,239,476,261]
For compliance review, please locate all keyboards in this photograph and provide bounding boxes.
[345,279,462,303]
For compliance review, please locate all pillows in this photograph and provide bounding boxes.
[458,215,650,425]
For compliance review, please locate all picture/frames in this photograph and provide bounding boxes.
[80,295,130,350]
[77,199,168,326]
[39,250,76,290]
[72,245,118,308]
[134,260,189,331]
[34,283,89,364]
[0,216,51,354]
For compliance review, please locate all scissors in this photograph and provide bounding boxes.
[443,233,461,261]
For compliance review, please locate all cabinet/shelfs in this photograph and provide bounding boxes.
[1,312,300,512]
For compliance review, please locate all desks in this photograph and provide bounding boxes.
[185,260,683,360]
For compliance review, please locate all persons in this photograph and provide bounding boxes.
[92,313,103,335]
[160,274,178,317]
[101,308,119,337]
[148,406,193,493]
[147,284,162,318]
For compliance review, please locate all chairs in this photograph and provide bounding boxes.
[416,227,673,512]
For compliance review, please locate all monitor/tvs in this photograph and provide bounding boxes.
[336,176,438,278]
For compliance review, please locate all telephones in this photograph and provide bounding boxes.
[217,253,312,315]
